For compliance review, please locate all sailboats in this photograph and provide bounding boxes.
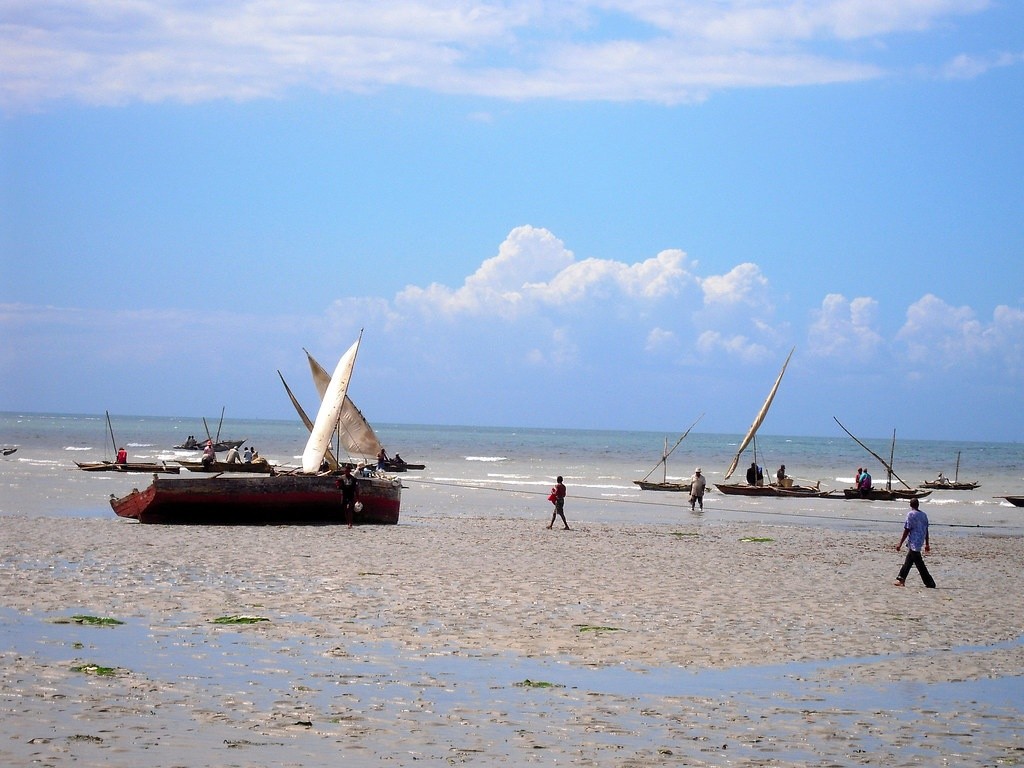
[277,348,427,474]
[713,345,837,498]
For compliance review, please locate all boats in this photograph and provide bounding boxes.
[834,416,933,501]
[168,460,279,474]
[992,494,1024,507]
[71,410,181,478]
[632,412,711,492]
[918,451,981,491]
[0,448,18,458]
[110,474,403,525]
[173,439,247,453]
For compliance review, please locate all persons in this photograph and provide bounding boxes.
[689,468,706,511]
[336,464,359,529]
[855,467,871,490]
[184,435,197,448]
[225,446,242,464]
[777,464,788,487]
[376,449,389,469]
[747,463,763,486]
[117,447,127,464]
[202,441,217,462]
[893,498,936,588]
[244,447,258,463]
[353,462,366,478]
[393,452,404,463]
[546,475,569,530]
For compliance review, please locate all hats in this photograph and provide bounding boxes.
[357,462,365,468]
[695,468,701,473]
[207,441,212,445]
[234,446,239,451]
